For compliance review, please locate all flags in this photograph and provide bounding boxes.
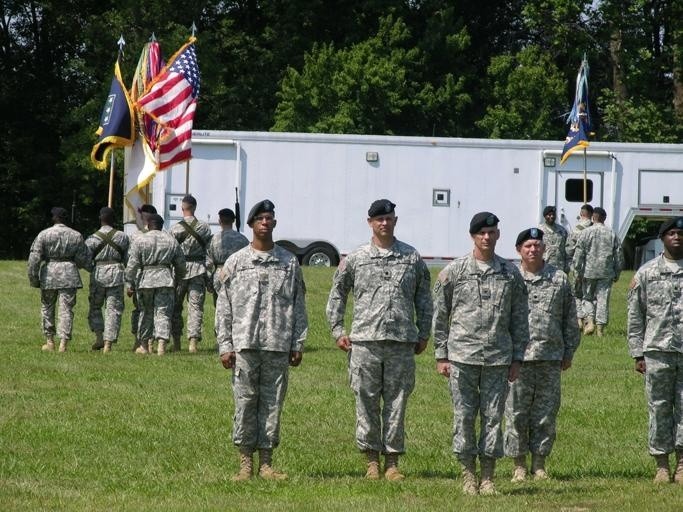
[92,35,201,233]
[560,60,593,164]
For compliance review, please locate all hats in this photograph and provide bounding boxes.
[659,216,683,238]
[247,199,274,224]
[543,206,556,217]
[218,209,236,219]
[182,195,196,205]
[140,205,156,213]
[582,204,593,214]
[469,212,499,233]
[100,207,115,217]
[592,207,606,216]
[51,206,70,218]
[516,228,543,246]
[147,214,164,224]
[368,199,396,217]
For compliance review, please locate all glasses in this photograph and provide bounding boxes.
[252,216,274,223]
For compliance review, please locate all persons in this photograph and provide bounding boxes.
[325,198,433,480]
[502,228,581,484]
[214,199,308,483]
[535,205,624,339]
[28,195,250,355]
[627,218,682,484]
[432,211,530,499]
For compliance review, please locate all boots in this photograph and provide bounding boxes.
[478,459,500,496]
[596,325,604,337]
[383,454,406,483]
[59,339,68,353]
[157,339,168,356]
[653,454,670,488]
[531,454,550,480]
[148,338,154,353]
[189,338,199,354]
[673,454,683,484]
[135,341,149,354]
[103,341,113,355]
[232,450,253,482]
[511,455,528,483]
[169,335,180,353]
[463,462,480,498]
[41,334,55,352]
[257,449,290,482]
[92,330,104,350]
[584,315,595,335]
[578,318,583,331]
[365,448,380,482]
